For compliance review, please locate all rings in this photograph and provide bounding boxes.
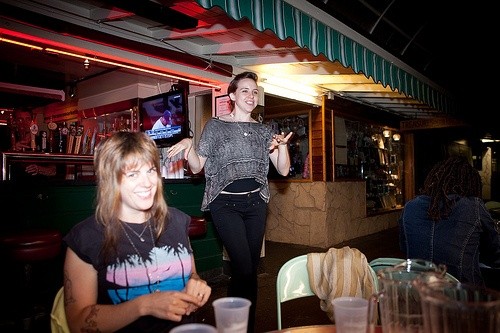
[198,292,204,297]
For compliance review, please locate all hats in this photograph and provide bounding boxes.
[163,110,172,125]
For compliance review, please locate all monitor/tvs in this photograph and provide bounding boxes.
[139,87,189,142]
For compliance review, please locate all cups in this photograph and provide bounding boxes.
[212,298,251,333]
[169,323,218,333]
[331,297,368,333]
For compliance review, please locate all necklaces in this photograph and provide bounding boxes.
[117,215,150,242]
[231,112,252,136]
[118,221,160,294]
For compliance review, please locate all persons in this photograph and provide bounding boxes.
[151,111,176,132]
[2,108,57,177]
[63,132,215,333]
[167,72,293,333]
[402,153,500,291]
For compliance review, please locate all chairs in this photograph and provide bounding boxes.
[275,251,381,331]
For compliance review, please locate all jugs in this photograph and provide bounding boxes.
[420,281,500,333]
[377,259,446,332]
